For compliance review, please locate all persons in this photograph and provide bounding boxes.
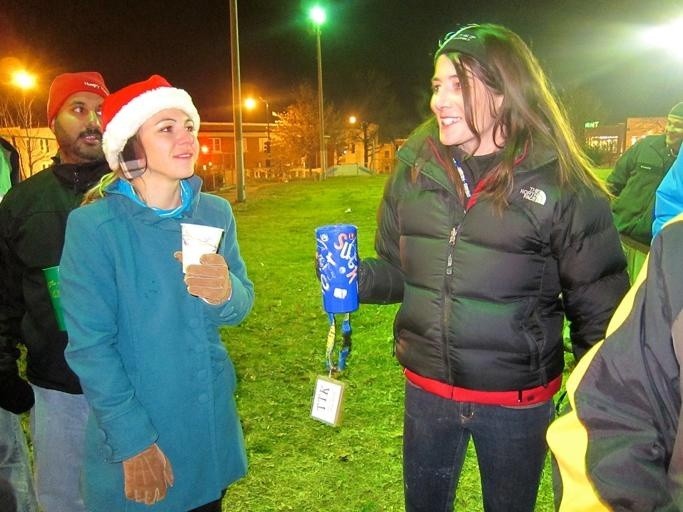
[0,135,42,511]
[0,71,113,511]
[545,214,682,510]
[315,21,632,510]
[61,72,257,511]
[646,136,682,241]
[604,99,682,284]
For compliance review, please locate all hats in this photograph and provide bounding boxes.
[46,71,201,171]
[668,102,683,118]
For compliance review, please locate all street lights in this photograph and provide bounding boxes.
[308,6,327,184]
[245,96,271,168]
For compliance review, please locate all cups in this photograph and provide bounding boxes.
[42,265,67,331]
[315,224,359,313]
[180,223,224,274]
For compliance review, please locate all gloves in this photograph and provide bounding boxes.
[174,251,232,304]
[123,443,174,505]
